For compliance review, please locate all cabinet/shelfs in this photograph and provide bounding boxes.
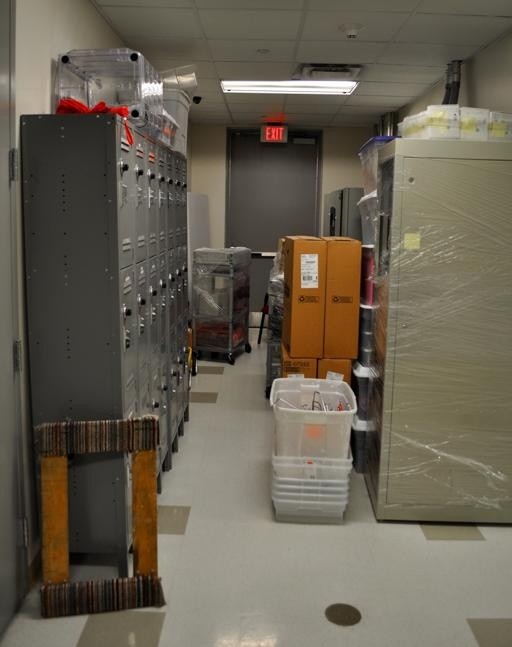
[15,104,195,581]
[370,143,511,527]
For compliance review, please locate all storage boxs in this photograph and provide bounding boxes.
[269,191,383,526]
